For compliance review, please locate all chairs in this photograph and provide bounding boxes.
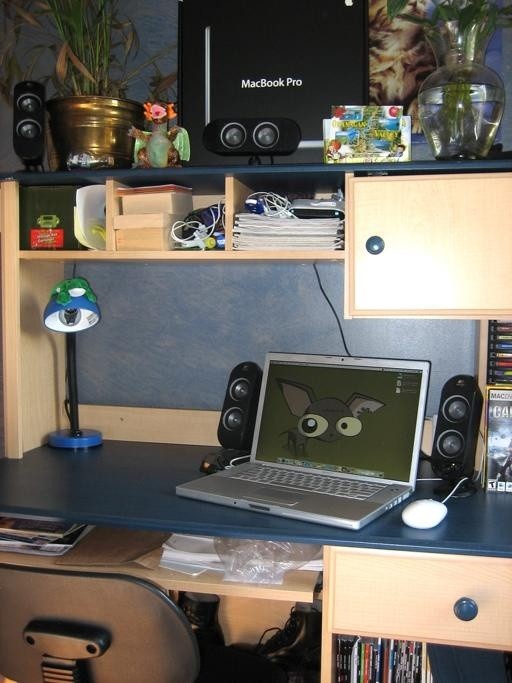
[0,564,204,683]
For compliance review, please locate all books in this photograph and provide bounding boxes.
[159,533,323,578]
[232,211,345,253]
[1,516,95,557]
[330,635,431,683]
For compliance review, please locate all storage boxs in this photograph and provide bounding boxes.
[121,192,194,215]
[114,215,174,251]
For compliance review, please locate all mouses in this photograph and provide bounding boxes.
[400,499,449,530]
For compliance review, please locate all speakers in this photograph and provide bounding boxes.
[199,360,263,474]
[430,373,484,494]
[203,116,301,155]
[13,81,46,165]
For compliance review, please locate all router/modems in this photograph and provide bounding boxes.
[288,199,344,220]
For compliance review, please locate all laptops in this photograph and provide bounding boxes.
[174,350,430,532]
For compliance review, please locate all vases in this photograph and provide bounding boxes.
[146,118,172,168]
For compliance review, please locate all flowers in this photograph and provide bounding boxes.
[139,97,176,122]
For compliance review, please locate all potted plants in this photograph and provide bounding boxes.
[0,0,177,167]
[387,0,512,158]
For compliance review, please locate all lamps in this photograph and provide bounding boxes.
[42,278,103,449]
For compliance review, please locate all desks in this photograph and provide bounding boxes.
[0,159,512,683]
